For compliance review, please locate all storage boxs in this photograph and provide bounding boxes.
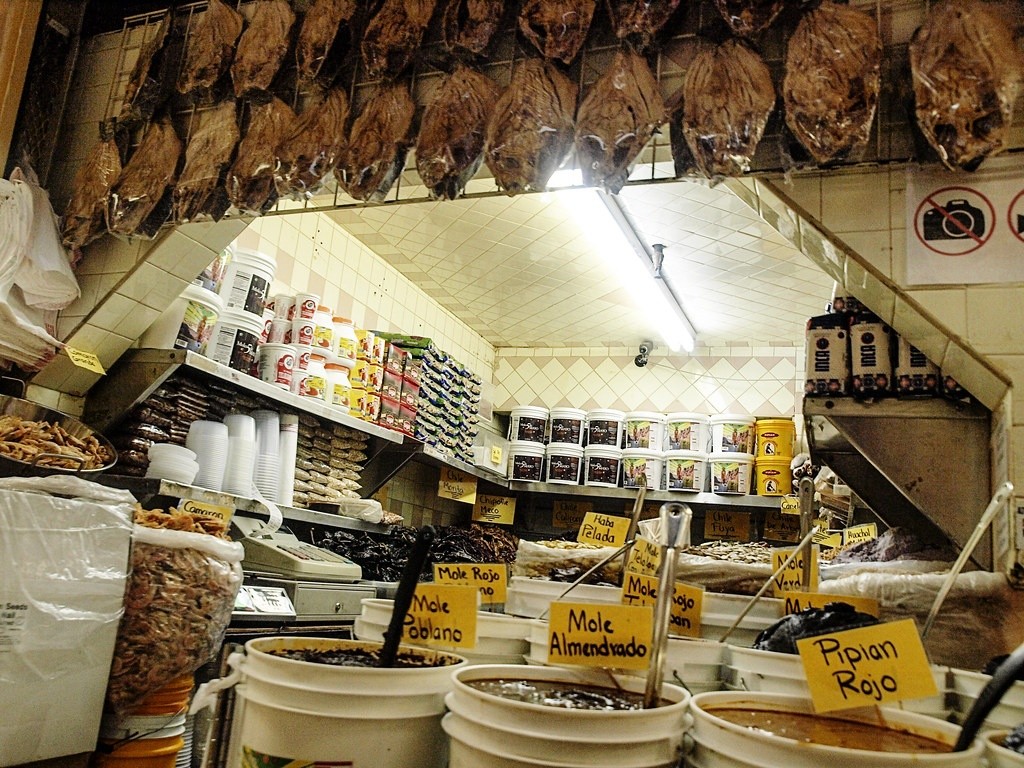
[382,342,405,376]
[354,329,375,361]
[400,378,419,412]
[378,396,400,430]
[381,370,403,403]
[404,360,422,387]
[349,360,369,390]
[366,393,381,423]
[348,389,367,419]
[398,403,416,437]
[367,364,383,397]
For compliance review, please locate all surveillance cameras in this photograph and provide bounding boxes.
[639,342,653,355]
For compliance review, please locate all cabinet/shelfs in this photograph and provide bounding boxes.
[78,347,992,574]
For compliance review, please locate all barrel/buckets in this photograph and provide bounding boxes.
[0,376,119,479]
[504,406,794,499]
[224,576,1024,768]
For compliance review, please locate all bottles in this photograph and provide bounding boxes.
[173,239,385,423]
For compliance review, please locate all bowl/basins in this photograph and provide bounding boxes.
[184,408,298,508]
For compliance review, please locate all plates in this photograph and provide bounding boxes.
[143,443,199,486]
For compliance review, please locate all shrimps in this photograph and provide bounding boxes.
[108,506,243,716]
[0,414,111,470]
